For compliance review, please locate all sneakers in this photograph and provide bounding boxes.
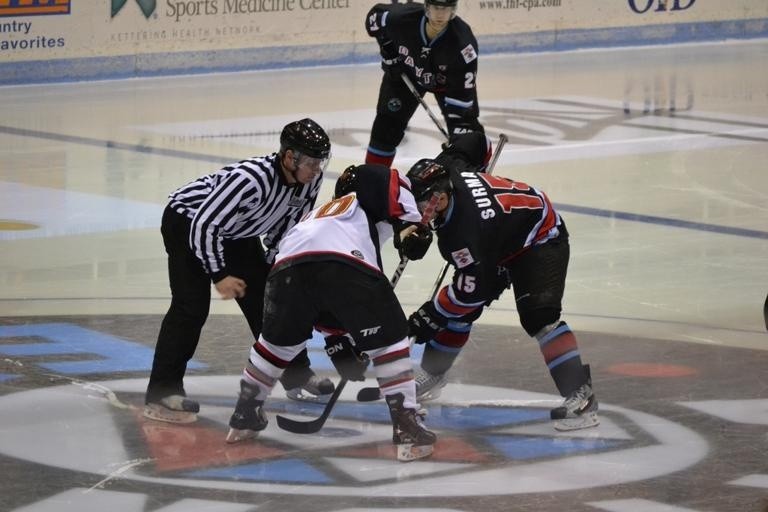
[145,396,199,412]
[230,396,268,431]
[393,418,435,445]
[416,370,447,397]
[283,370,334,395]
[551,386,598,419]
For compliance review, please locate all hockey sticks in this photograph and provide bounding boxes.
[276,192,441,434]
[357,134,508,401]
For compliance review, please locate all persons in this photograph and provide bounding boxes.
[229,161,437,446]
[145,118,335,414]
[364,0,480,166]
[405,116,594,421]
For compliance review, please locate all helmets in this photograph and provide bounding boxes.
[335,165,359,197]
[281,118,330,174]
[423,0,458,20]
[406,158,453,229]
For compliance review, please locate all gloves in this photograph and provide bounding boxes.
[407,300,446,344]
[378,54,405,78]
[397,222,432,260]
[323,336,368,379]
